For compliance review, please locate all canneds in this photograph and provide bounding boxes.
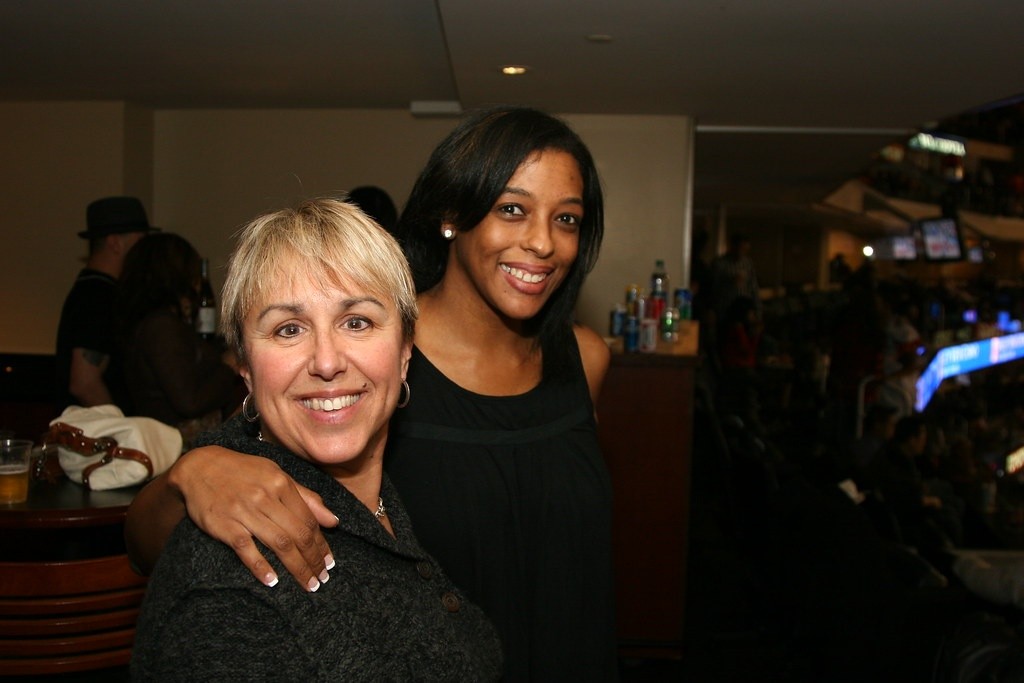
[609,282,691,354]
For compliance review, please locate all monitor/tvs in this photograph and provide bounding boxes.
[888,233,918,264]
[914,213,966,265]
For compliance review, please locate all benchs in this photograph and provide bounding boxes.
[0,352,150,683]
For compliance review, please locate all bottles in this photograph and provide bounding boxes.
[652,258,668,304]
[195,256,216,338]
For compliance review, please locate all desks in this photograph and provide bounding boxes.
[0,477,141,561]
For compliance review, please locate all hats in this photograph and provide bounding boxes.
[76,195,163,239]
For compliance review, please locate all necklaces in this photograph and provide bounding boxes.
[374,497,386,519]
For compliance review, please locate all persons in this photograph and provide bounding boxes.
[55,196,245,427]
[344,186,427,295]
[123,106,620,683]
[129,194,505,683]
[695,217,1024,625]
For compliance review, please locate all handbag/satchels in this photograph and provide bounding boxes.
[41,403,183,491]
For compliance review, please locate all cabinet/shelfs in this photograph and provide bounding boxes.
[597,364,697,661]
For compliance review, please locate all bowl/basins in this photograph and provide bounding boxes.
[0,439,34,475]
